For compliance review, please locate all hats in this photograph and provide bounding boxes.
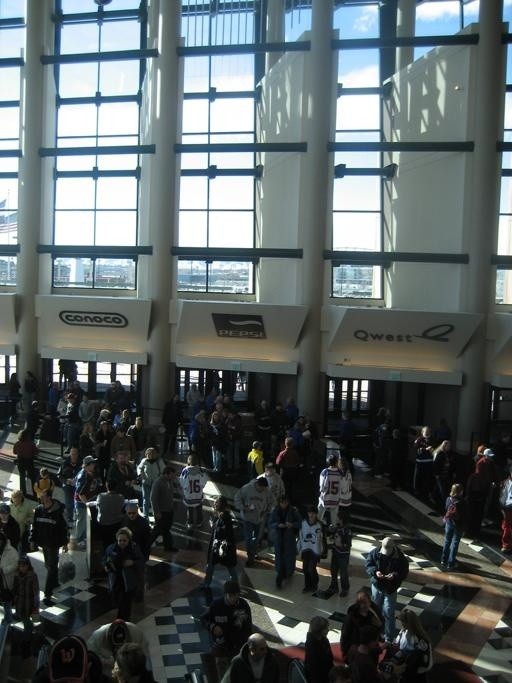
[355,585,372,600]
[483,448,495,457]
[252,440,263,448]
[326,454,339,466]
[450,483,464,497]
[83,455,99,465]
[379,536,396,557]
[47,634,88,681]
[109,619,129,656]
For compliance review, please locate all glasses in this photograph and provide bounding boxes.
[128,512,135,514]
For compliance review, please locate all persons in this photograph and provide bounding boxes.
[1,360,435,682]
[434,433,512,571]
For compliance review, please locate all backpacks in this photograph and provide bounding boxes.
[443,496,463,527]
